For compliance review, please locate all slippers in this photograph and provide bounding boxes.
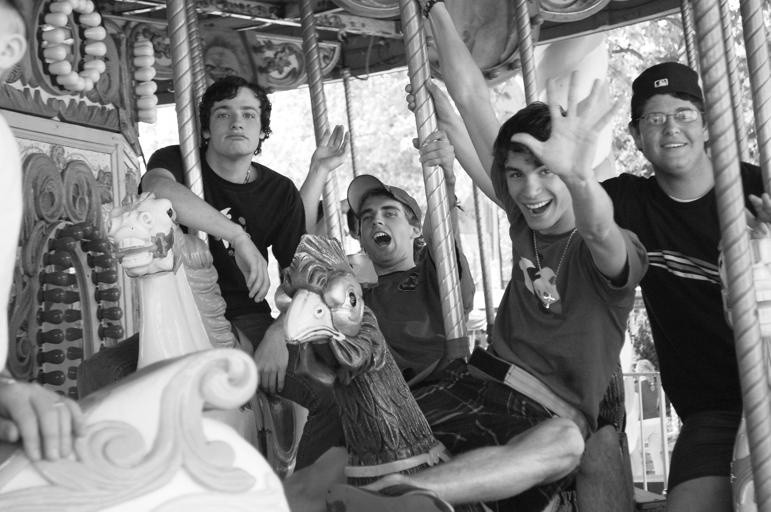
[326,483,455,512]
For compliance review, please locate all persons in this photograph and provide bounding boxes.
[139,75,339,471]
[0,2,91,464]
[598,63,767,510]
[406,63,771,512]
[301,124,473,396]
[363,0,648,505]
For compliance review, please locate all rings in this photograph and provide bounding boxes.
[52,400,65,408]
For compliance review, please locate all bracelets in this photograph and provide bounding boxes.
[421,1,442,15]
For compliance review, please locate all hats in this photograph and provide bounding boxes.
[348,174,422,224]
[631,62,703,103]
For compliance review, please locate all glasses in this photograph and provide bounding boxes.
[635,110,705,125]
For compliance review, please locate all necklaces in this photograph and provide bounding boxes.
[531,228,579,307]
[244,166,255,186]
